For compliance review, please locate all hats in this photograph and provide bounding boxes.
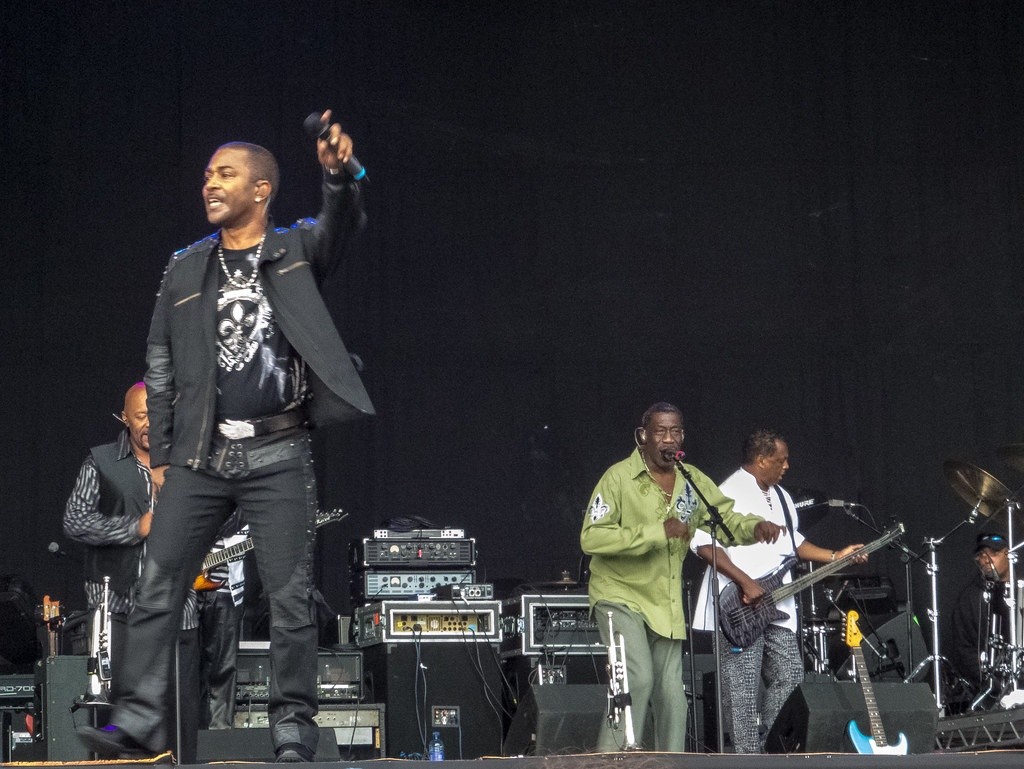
[974,532,1008,554]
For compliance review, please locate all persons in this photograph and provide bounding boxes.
[951,531,1010,691]
[63,380,204,732]
[580,400,781,753]
[74,108,375,755]
[689,428,869,755]
[199,522,251,731]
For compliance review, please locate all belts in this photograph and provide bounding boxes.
[213,410,304,440]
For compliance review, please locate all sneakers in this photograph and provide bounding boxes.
[275,742,315,763]
[76,723,158,759]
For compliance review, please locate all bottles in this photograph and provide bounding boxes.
[428,732,444,760]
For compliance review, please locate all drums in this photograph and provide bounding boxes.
[982,578,1024,676]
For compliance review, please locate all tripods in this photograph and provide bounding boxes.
[904,498,989,718]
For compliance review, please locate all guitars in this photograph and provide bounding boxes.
[191,508,351,589]
[842,610,908,756]
[24,596,60,739]
[717,514,907,648]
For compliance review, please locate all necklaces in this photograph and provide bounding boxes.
[641,451,672,498]
[218,231,266,288]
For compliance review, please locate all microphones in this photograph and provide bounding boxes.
[49,542,69,557]
[827,499,857,508]
[665,449,686,461]
[302,112,369,185]
[990,557,1000,582]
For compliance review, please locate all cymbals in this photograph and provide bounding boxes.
[997,443,1024,472]
[942,460,1021,526]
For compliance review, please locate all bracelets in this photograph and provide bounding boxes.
[323,163,343,175]
[831,550,836,561]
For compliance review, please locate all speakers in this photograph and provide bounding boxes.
[835,610,930,683]
[361,642,503,760]
[503,683,655,758]
[765,681,938,756]
[31,655,97,762]
[197,727,340,764]
[505,656,612,740]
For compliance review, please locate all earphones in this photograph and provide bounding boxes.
[640,433,647,444]
[681,430,685,442]
